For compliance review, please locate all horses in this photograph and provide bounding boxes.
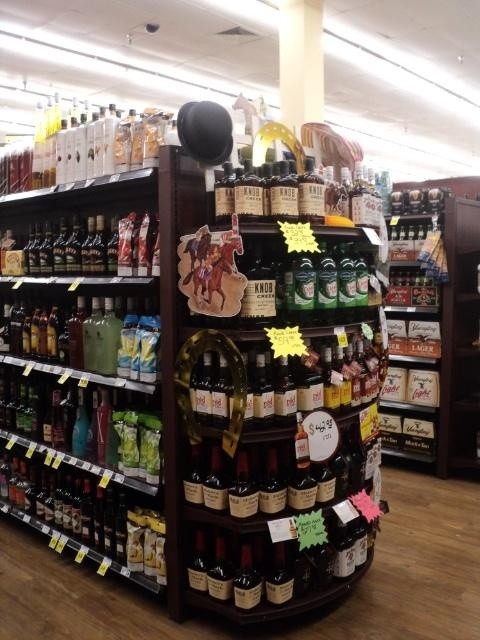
[182,233,243,311]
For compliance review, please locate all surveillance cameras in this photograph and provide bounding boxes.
[145,24,160,34]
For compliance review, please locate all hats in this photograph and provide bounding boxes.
[300,119,364,184]
[177,100,233,165]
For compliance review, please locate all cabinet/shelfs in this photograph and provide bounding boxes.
[1,143,388,636]
[380,177,479,487]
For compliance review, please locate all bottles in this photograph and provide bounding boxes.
[0,448,128,564]
[21,213,122,276]
[190,341,385,423]
[182,423,384,515]
[0,293,157,378]
[0,370,121,470]
[182,525,299,615]
[236,239,383,324]
[329,510,376,586]
[387,224,442,241]
[0,102,162,199]
[214,155,382,229]
[388,270,435,286]
